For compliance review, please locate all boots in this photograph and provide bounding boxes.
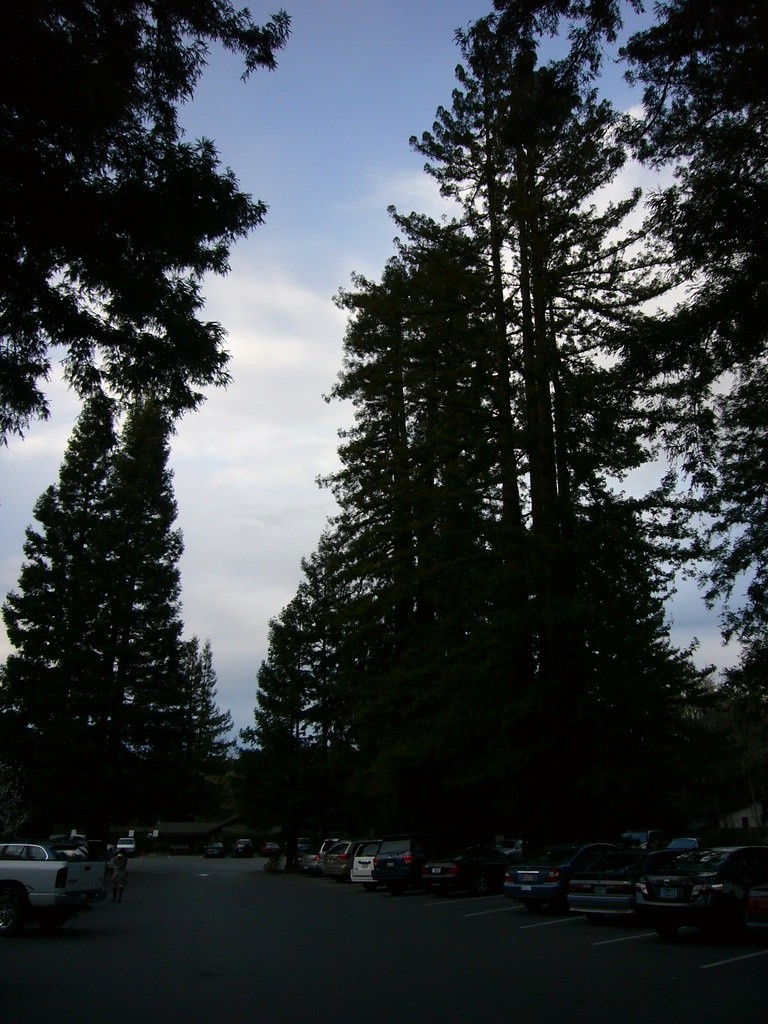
[117,887,124,903]
[112,887,117,902]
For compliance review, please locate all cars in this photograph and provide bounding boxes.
[298,834,513,896]
[502,829,768,932]
[493,839,528,862]
[0,835,117,935]
[233,839,253,857]
[117,838,135,852]
[261,842,280,853]
[204,842,224,854]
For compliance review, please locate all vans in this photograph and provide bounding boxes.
[297,838,312,852]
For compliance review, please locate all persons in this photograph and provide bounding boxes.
[111,848,128,904]
